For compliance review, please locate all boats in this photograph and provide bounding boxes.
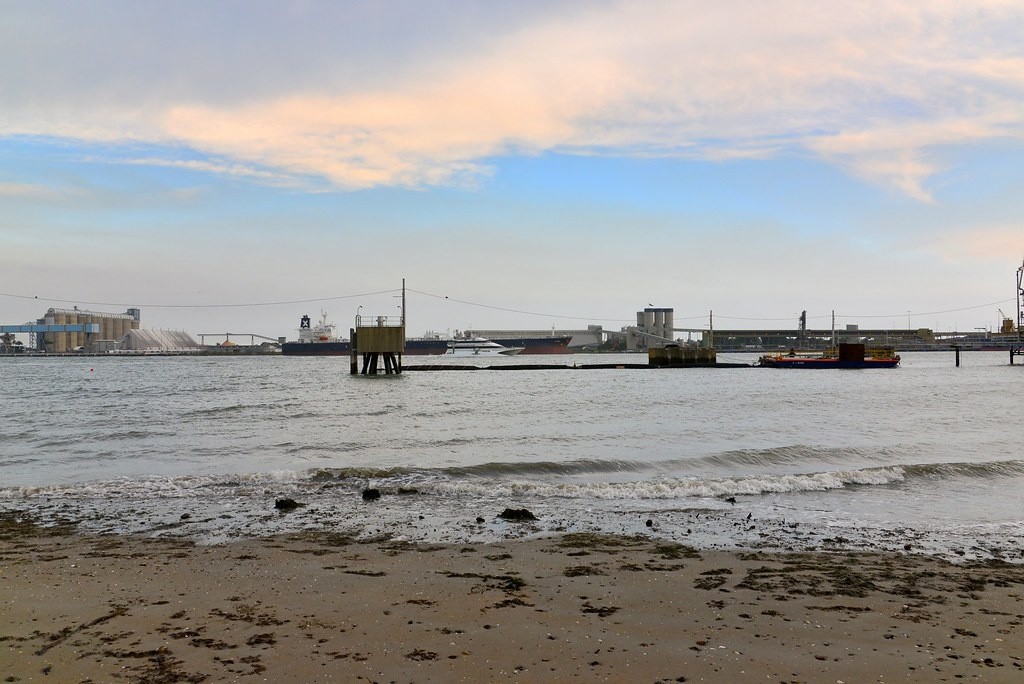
[445,332,526,357]
[281,279,573,355]
[758,309,902,368]
